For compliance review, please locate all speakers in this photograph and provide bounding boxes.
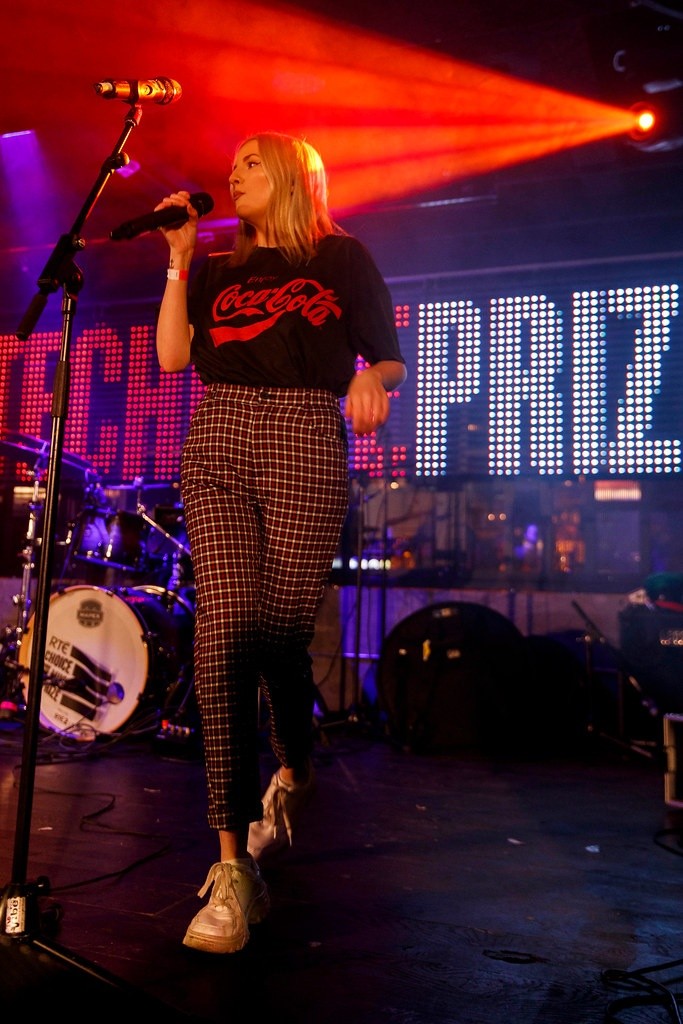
[615,609,683,771]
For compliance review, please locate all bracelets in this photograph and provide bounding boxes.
[165,268,190,281]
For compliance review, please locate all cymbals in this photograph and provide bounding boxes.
[0,430,89,473]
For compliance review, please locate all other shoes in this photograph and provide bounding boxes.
[184,862,265,953]
[246,772,302,862]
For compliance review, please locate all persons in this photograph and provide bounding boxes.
[153,133,408,954]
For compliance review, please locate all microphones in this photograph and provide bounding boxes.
[109,192,214,240]
[52,679,85,693]
[92,78,183,105]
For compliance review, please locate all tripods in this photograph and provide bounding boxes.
[0,105,193,1024]
[319,487,373,730]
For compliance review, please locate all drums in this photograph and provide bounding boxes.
[15,579,201,747]
[67,500,152,576]
